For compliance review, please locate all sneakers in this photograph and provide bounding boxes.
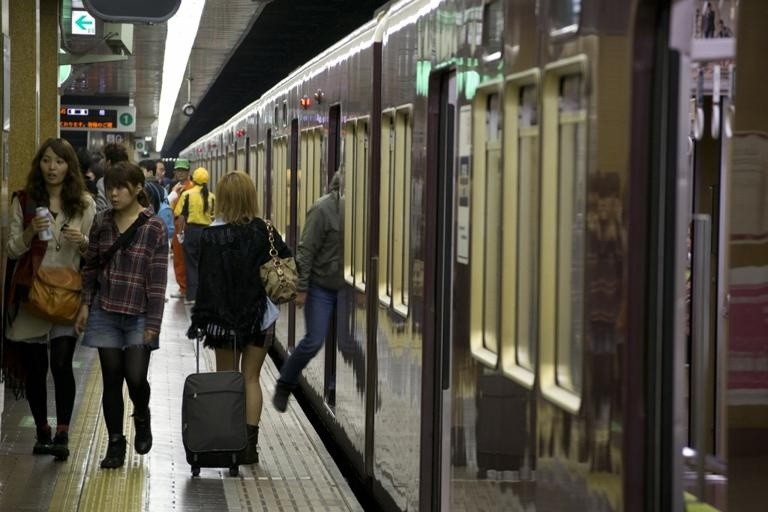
[134,408,152,454]
[33,431,68,456]
[101,440,126,468]
[274,380,290,412]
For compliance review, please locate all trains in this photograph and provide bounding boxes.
[178,1,768,512]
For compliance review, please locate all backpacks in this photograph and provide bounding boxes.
[150,182,174,238]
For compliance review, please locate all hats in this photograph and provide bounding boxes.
[174,160,209,185]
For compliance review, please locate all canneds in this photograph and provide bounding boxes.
[35,206,53,241]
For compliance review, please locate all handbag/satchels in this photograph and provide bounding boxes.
[27,267,82,324]
[259,218,299,305]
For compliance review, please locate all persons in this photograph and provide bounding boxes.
[0,137,96,460]
[273,172,346,423]
[72,161,161,471]
[38,131,258,308]
[177,169,296,465]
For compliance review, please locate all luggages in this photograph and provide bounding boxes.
[182,334,248,476]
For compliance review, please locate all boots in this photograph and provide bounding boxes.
[246,424,259,463]
